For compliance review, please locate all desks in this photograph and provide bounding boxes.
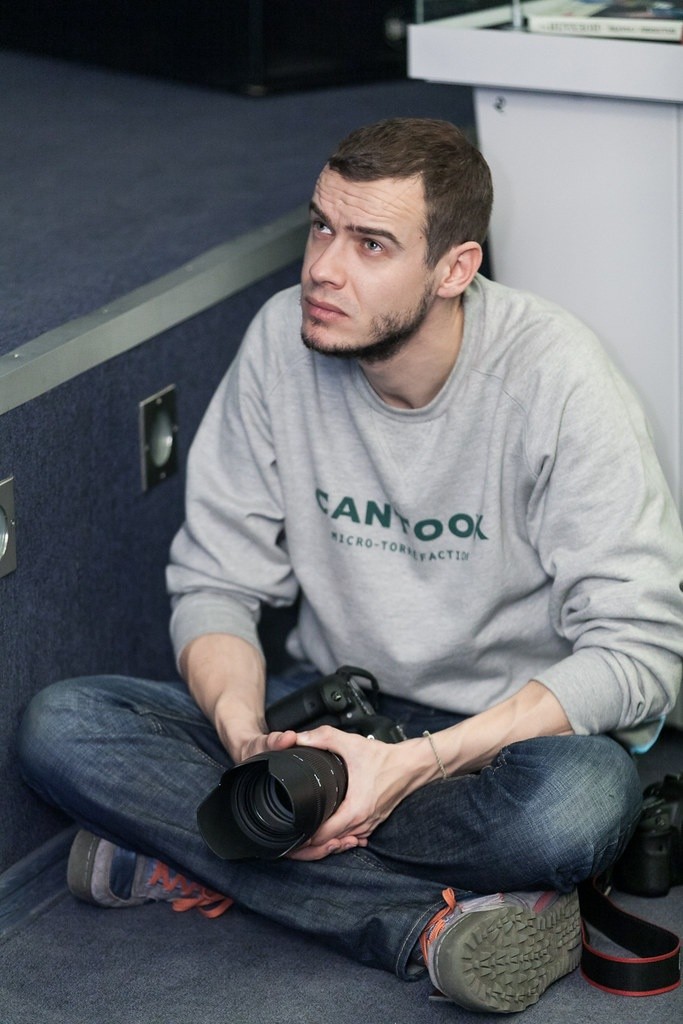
[408,0,683,513]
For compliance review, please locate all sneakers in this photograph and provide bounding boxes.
[419,887,584,1014]
[66,827,235,918]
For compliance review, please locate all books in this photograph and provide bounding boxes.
[530,0,683,44]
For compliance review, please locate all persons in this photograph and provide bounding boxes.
[12,119,683,1013]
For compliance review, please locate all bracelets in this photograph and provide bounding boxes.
[423,728,447,781]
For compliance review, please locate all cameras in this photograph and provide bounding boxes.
[196,673,416,862]
[606,773,683,897]
